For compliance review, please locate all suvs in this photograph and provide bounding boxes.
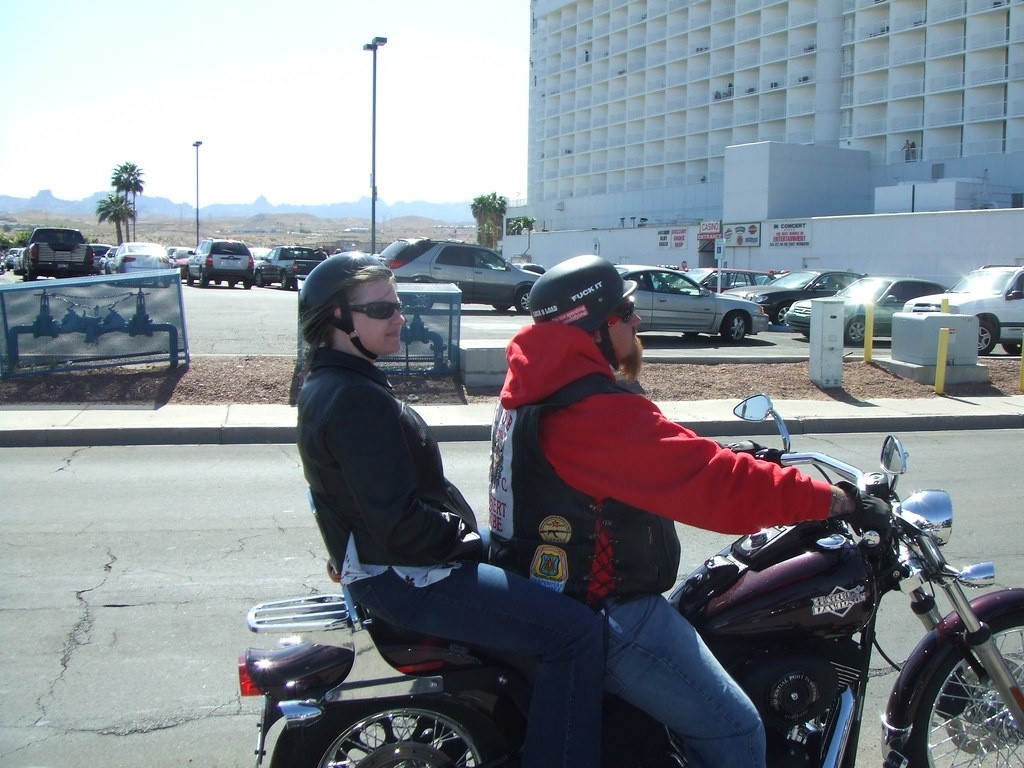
[185,237,256,289]
[376,236,543,315]
[903,264,1023,355]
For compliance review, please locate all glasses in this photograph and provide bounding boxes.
[612,297,636,321]
[341,300,403,319]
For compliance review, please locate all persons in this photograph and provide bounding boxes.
[297,251,606,768]
[900,140,916,163]
[488,254,892,768]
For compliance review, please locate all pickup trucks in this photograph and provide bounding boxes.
[23,227,95,281]
[253,246,325,291]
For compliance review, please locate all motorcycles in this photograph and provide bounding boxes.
[237,391,1024,768]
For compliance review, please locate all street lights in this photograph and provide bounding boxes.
[192,140,203,247]
[363,36,387,254]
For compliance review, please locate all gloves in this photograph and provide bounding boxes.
[834,481,892,536]
[714,439,767,458]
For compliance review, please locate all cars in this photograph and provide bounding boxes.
[0,246,26,275]
[87,242,196,286]
[608,264,949,346]
[510,263,546,274]
[246,247,272,270]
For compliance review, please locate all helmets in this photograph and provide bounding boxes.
[529,254,638,335]
[297,251,385,318]
